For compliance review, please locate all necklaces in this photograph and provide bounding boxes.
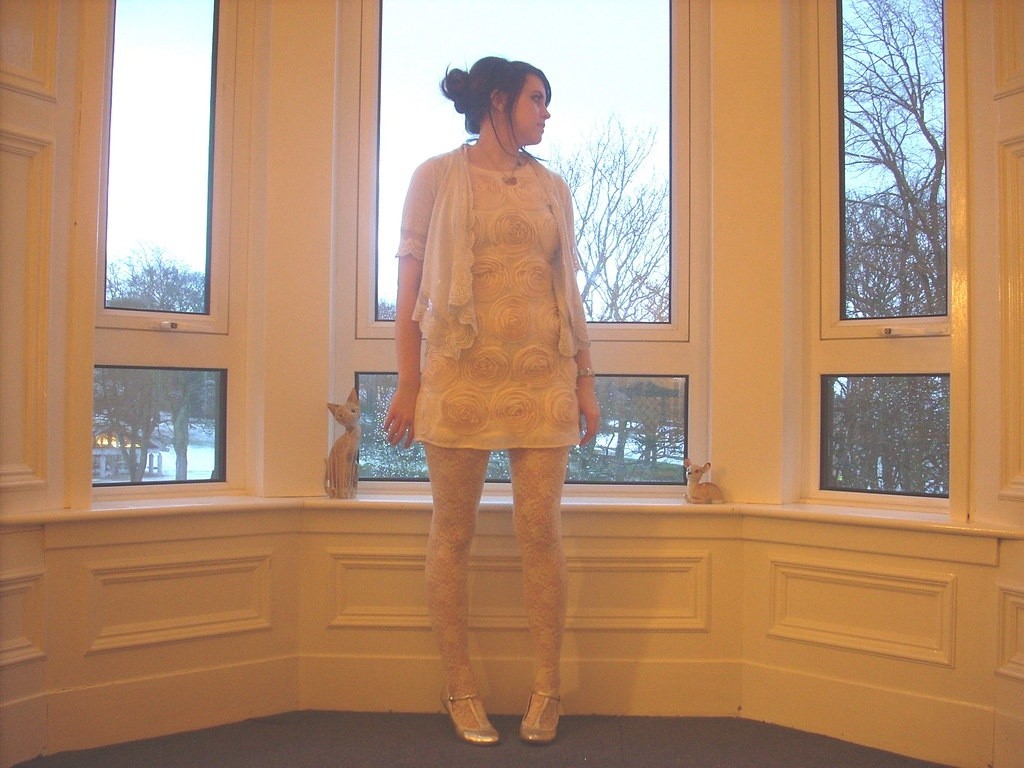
[475,139,522,186]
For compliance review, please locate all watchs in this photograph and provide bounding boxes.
[577,367,595,378]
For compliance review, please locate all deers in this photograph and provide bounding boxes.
[682,457,723,504]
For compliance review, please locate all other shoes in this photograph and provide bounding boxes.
[441,680,500,746]
[519,688,561,744]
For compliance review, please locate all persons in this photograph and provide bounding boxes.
[384,56,601,744]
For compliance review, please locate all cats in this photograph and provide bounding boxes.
[322,387,361,499]
